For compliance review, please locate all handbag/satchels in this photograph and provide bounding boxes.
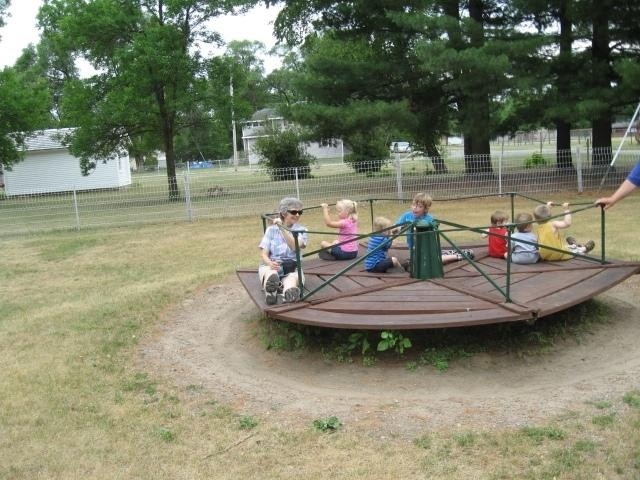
[283,260,296,275]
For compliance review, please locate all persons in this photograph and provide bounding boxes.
[392,192,475,265]
[258,196,307,305]
[503,213,541,264]
[317,199,359,261]
[365,216,409,274]
[533,201,596,262]
[593,118,640,212]
[481,211,509,260]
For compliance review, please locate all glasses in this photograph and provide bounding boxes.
[287,210,302,215]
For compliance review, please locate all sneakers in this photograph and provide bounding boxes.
[319,251,336,261]
[567,237,576,244]
[283,288,299,302]
[585,240,594,252]
[442,249,473,260]
[266,272,279,304]
